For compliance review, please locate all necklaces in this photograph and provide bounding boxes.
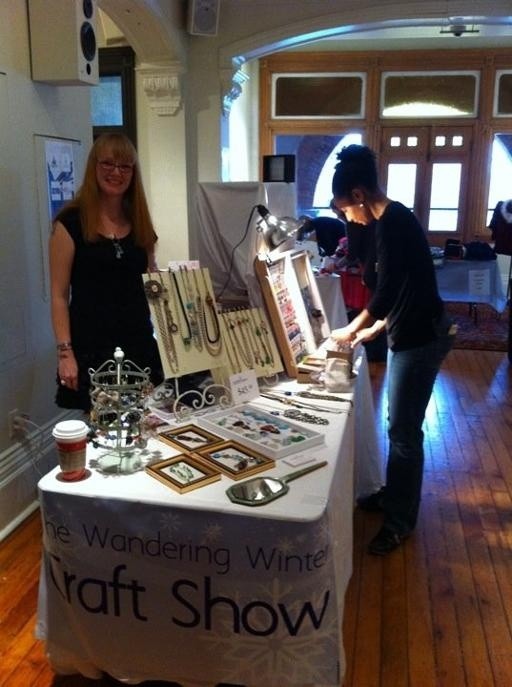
[145,265,274,374]
[499,200,511,224]
[244,386,354,424]
[100,216,129,259]
[328,143,454,553]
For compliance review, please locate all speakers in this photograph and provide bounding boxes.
[186,0,220,37]
[28,0,100,87]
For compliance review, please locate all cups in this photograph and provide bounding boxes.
[52,419,92,481]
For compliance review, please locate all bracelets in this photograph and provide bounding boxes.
[56,342,73,347]
[217,409,289,439]
[370,325,381,335]
[60,348,73,352]
[49,132,166,411]
[59,354,71,358]
[169,461,193,478]
[212,452,254,469]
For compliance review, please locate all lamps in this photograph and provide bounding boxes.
[215,204,306,304]
[439,0,480,37]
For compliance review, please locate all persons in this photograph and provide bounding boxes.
[487,199,512,256]
[326,197,386,361]
[299,213,345,256]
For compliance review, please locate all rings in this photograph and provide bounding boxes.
[61,378,69,385]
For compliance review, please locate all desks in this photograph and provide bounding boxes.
[37,327,392,685]
[429,245,505,330]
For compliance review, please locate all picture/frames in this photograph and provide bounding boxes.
[140,397,330,496]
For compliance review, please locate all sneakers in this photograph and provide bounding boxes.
[359,494,407,555]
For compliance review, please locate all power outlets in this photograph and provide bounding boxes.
[8,408,22,441]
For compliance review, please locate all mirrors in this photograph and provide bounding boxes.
[225,460,329,506]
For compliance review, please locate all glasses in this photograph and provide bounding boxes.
[100,161,131,173]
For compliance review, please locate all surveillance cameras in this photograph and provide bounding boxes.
[450,26,465,36]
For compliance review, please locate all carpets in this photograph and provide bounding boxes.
[443,301,511,353]
